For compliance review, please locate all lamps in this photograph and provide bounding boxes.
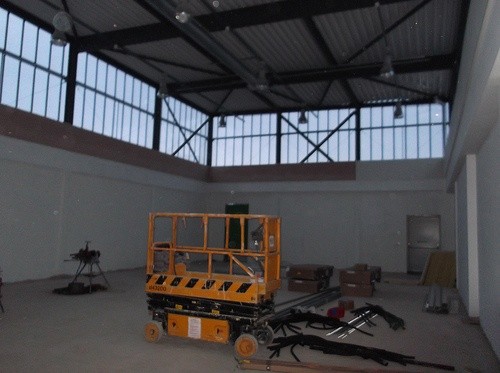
[175,0,190,24]
[49,12,72,45]
[219,111,227,127]
[156,79,168,97]
[380,49,395,79]
[393,85,403,120]
[255,71,269,89]
[299,107,308,124]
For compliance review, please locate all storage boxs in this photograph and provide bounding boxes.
[339,264,381,297]
[286,263,334,293]
